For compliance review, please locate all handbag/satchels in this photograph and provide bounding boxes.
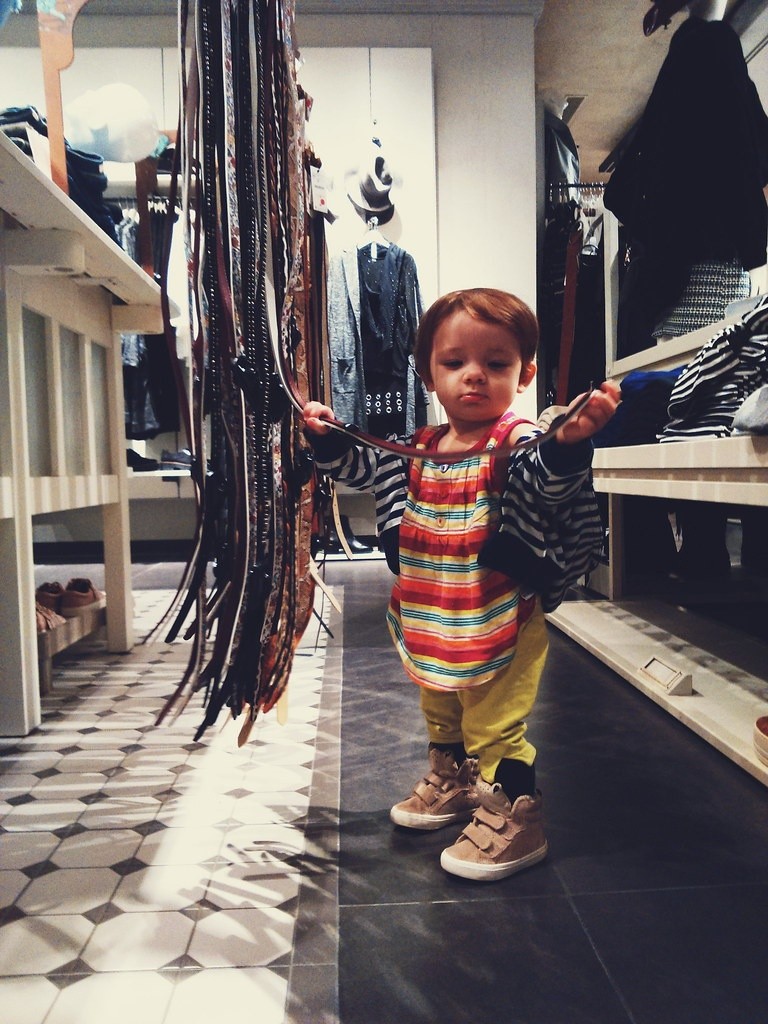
[364,377,409,418]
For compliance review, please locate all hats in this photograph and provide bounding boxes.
[342,152,398,213]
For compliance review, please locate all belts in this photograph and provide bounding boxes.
[141,0,329,751]
[555,221,586,407]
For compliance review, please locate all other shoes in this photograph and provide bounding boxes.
[753,714,768,769]
[158,447,194,470]
[127,448,160,471]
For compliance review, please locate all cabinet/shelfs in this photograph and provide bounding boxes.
[546,196,768,792]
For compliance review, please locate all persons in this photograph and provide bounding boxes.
[303,289,620,880]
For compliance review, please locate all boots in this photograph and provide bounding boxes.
[322,514,373,554]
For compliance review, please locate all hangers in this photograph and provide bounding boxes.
[546,179,606,205]
[358,219,389,253]
[114,196,194,210]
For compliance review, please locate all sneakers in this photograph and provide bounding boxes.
[439,774,550,882]
[35,581,67,614]
[388,745,482,830]
[59,575,106,618]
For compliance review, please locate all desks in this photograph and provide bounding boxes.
[0,129,179,727]
